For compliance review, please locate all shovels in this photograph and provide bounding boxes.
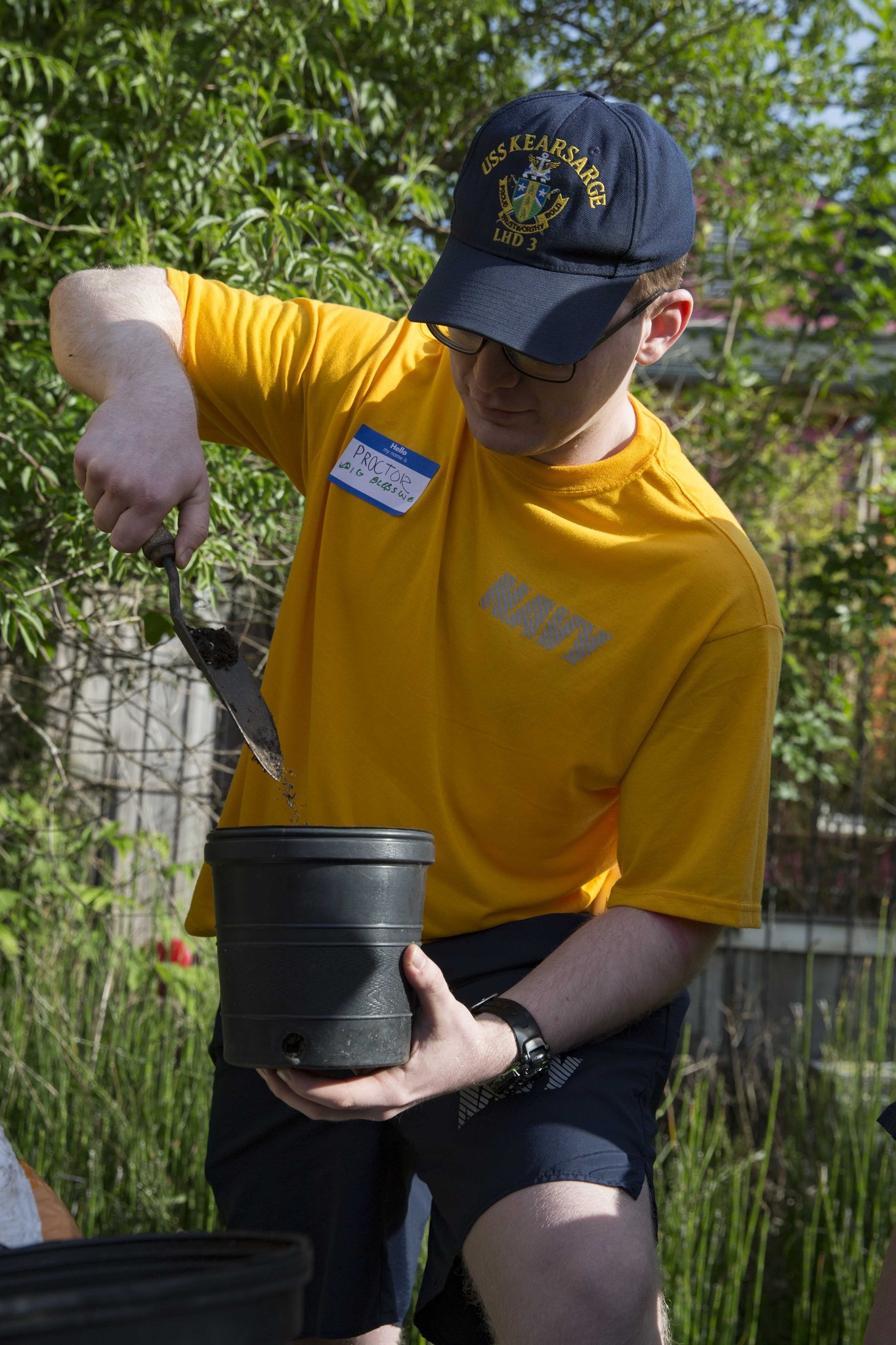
[145,519,294,783]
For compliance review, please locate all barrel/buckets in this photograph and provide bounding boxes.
[200,823,441,1078]
[0,1230,311,1345]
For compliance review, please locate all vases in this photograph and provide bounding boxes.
[204,824,436,1073]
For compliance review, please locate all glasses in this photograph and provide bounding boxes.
[424,288,671,385]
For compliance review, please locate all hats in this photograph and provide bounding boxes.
[407,90,697,365]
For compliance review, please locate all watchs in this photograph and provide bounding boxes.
[469,993,552,1096]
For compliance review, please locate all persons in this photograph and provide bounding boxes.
[49,91,785,1344]
